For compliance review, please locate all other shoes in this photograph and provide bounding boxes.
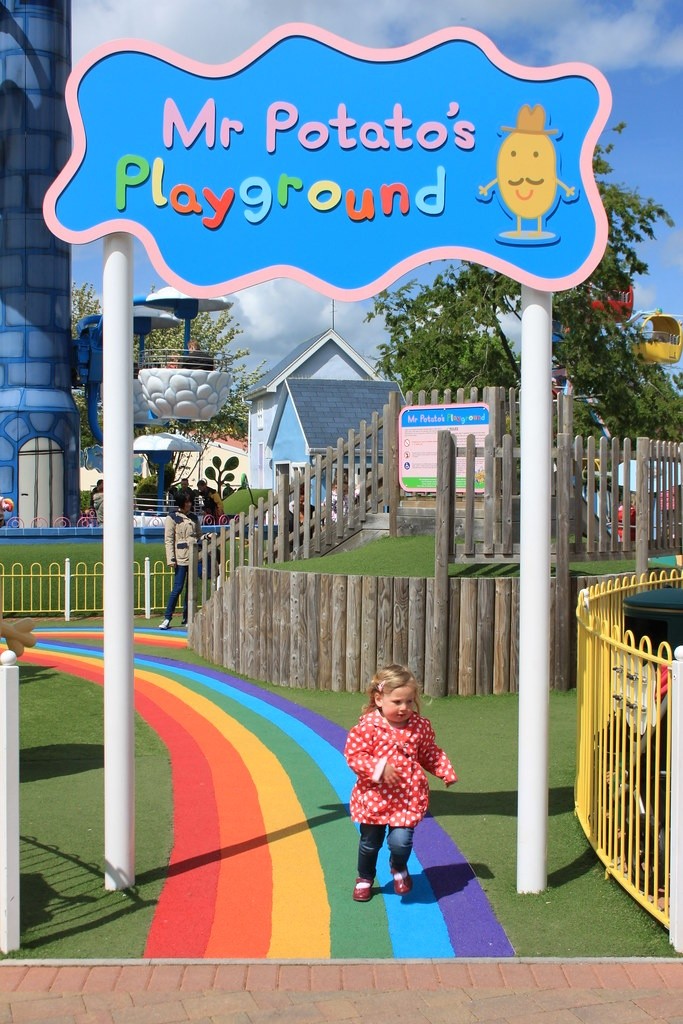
[353,877,374,902]
[182,618,188,624]
[159,625,167,629]
[391,866,411,894]
[186,624,188,627]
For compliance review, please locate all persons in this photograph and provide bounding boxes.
[91,479,224,524]
[617,486,675,541]
[165,342,214,370]
[287,469,358,552]
[158,490,216,629]
[345,665,457,901]
